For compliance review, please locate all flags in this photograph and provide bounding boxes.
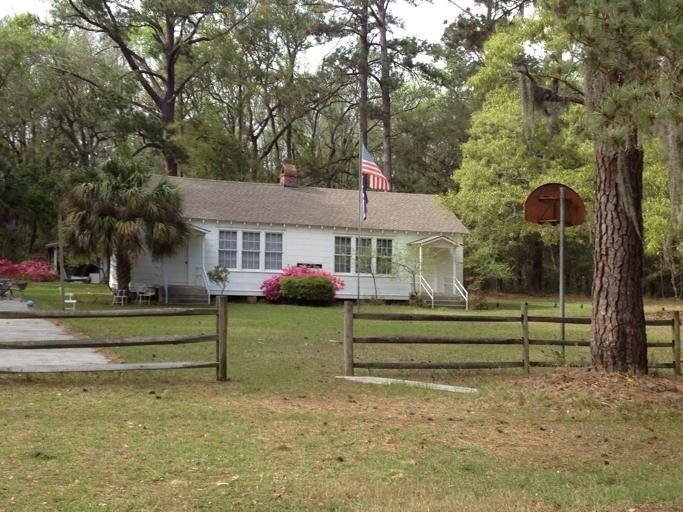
[360,141,390,192]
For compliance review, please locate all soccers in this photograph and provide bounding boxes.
[25,300,33,307]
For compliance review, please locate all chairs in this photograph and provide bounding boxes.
[111,281,155,306]
[64,293,77,312]
